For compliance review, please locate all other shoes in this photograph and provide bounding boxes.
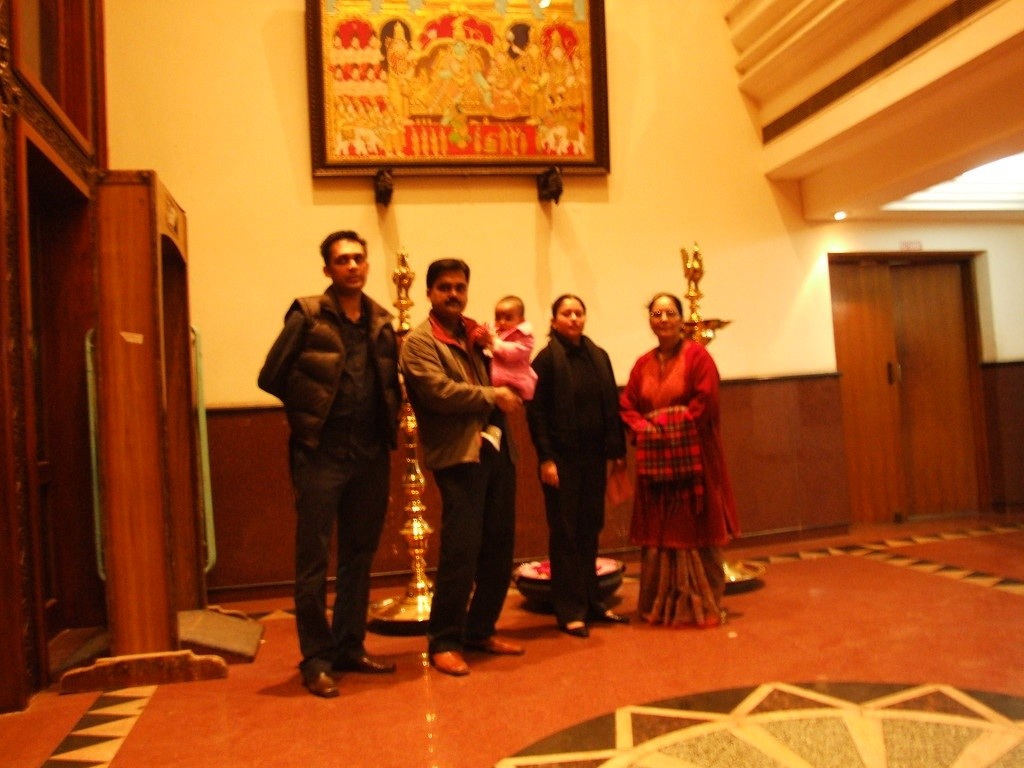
[427,650,468,677]
[587,608,630,625]
[467,635,524,655]
[562,617,587,637]
[479,431,501,452]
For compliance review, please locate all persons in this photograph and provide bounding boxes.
[619,292,740,626]
[525,295,628,638]
[403,259,537,674]
[474,296,537,455]
[258,232,402,696]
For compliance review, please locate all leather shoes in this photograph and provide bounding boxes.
[301,669,340,699]
[331,653,395,672]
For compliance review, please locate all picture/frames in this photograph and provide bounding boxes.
[305,0,612,177]
